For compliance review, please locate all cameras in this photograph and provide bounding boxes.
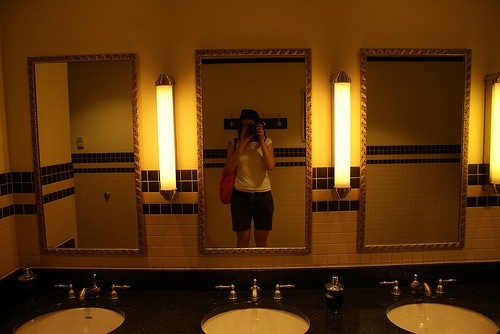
[245,124,258,135]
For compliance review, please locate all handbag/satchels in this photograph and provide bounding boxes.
[219,137,238,205]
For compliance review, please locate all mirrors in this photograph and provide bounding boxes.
[356,45,472,254]
[194,46,314,257]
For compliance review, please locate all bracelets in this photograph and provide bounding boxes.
[261,143,266,147]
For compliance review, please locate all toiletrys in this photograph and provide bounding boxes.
[16,266,42,304]
[324,275,345,311]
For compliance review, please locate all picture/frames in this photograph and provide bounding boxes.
[24,51,151,258]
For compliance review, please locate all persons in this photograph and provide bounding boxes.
[226,109,276,248]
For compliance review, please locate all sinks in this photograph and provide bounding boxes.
[385,300,499,334]
[13,306,125,334]
[201,302,311,334]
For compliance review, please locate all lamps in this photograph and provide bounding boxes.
[154,71,178,199]
[330,67,355,200]
[486,72,500,192]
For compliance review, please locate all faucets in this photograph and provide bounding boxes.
[409,273,432,297]
[78,273,102,302]
[249,277,263,303]
